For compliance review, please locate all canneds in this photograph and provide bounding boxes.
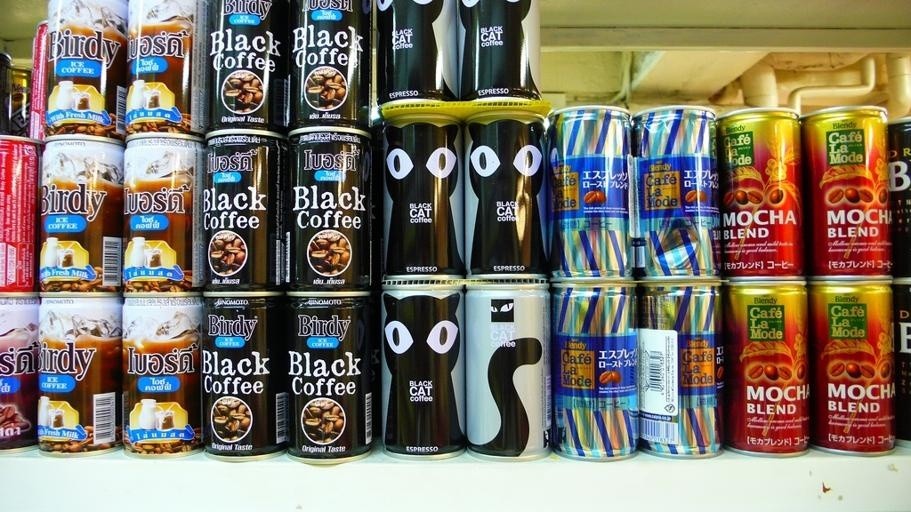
[715,104,898,459]
[0,0,555,467]
[887,116,911,441]
[551,102,730,460]
